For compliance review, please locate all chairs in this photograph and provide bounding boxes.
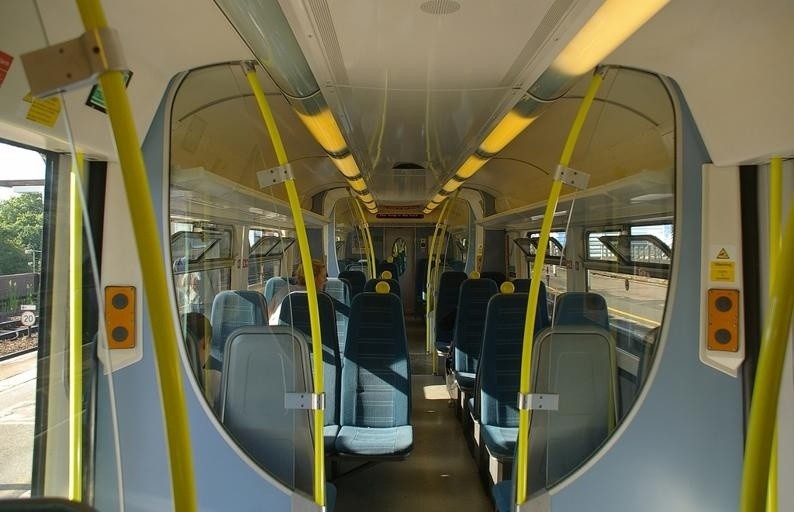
[277,290,341,450]
[181,256,611,371]
[335,292,416,463]
[451,276,502,415]
[467,328,486,421]
[492,326,622,500]
[479,292,544,464]
[213,327,339,500]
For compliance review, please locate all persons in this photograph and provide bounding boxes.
[182,257,214,314]
[268,258,327,328]
[182,311,222,411]
[174,240,195,320]
